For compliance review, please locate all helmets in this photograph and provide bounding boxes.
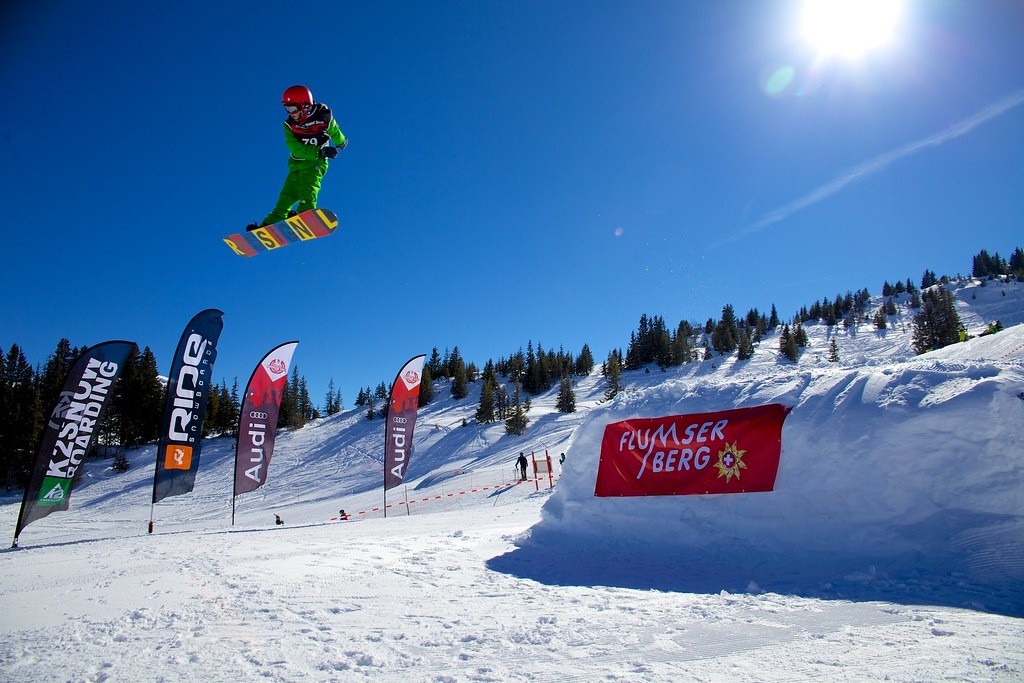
[282,85,313,123]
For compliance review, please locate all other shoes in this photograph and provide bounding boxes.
[288,210,298,218]
[246,221,267,231]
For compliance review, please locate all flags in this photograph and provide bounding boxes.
[236,343,298,497]
[22,343,134,530]
[156,312,224,503]
[386,356,426,492]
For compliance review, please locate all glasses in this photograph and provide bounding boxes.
[284,104,299,114]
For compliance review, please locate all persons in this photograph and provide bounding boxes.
[340,510,348,520]
[246,85,348,232]
[515,452,528,481]
[559,453,565,464]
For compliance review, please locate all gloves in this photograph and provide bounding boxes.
[336,138,348,150]
[319,147,338,159]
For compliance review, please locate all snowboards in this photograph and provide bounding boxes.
[221,207,338,258]
